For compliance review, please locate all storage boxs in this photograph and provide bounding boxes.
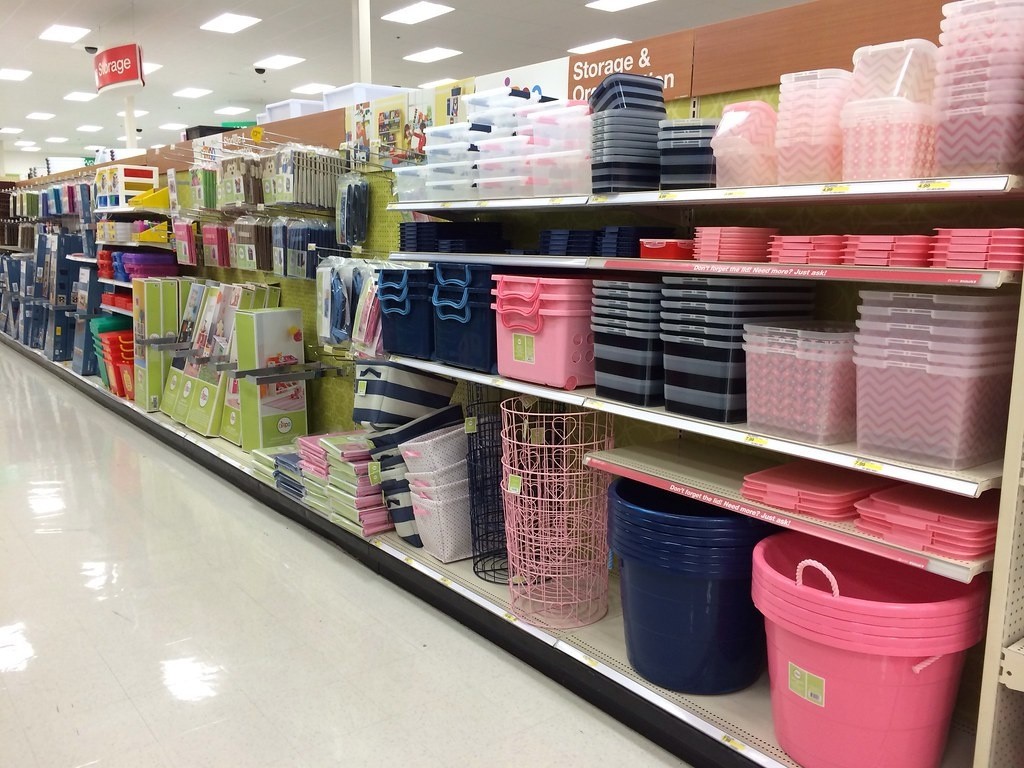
[1,234,308,454]
[350,0,1023,564]
[186,82,423,143]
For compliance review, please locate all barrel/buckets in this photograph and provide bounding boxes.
[606,476,768,696]
[750,530,989,768]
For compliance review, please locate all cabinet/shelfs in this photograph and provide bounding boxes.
[553,170,1024,768]
[367,194,587,650]
[93,207,176,318]
